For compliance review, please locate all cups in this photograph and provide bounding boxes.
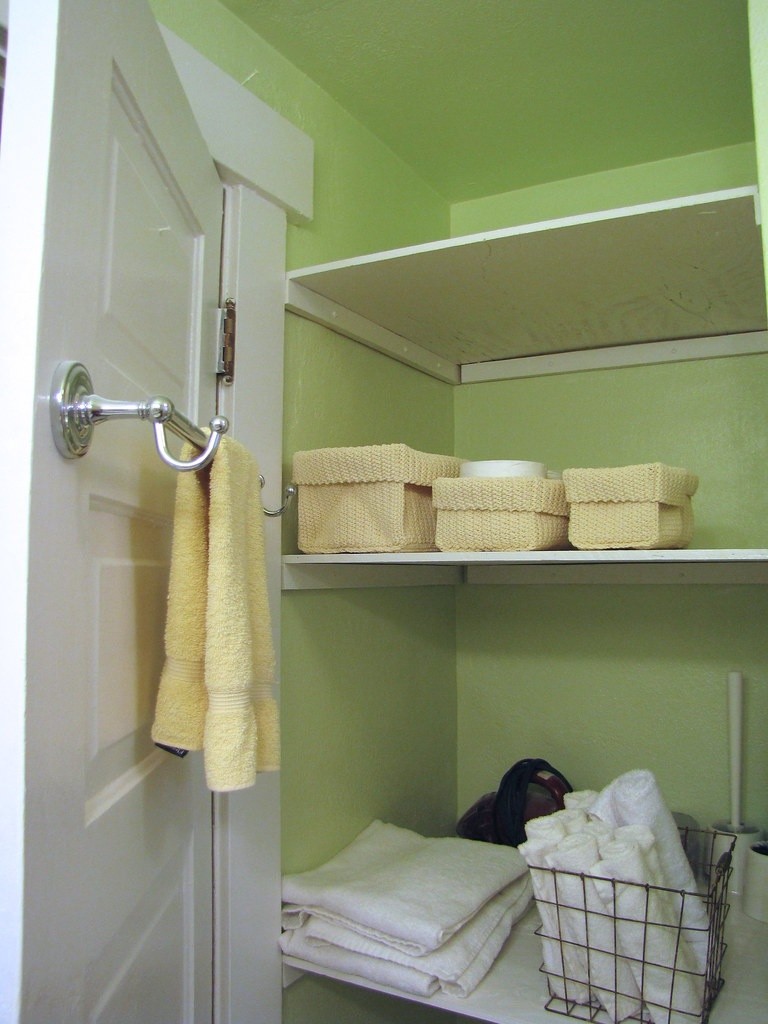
[741,840,768,923]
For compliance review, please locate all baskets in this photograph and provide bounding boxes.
[431,476,578,551]
[292,442,472,554]
[561,461,700,551]
[523,821,741,1023]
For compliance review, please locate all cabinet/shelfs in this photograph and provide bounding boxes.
[283,185,768,1022]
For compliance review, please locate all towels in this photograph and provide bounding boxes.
[148,426,278,792]
[277,820,544,999]
[517,768,716,1024]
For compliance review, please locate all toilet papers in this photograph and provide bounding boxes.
[459,460,544,478]
